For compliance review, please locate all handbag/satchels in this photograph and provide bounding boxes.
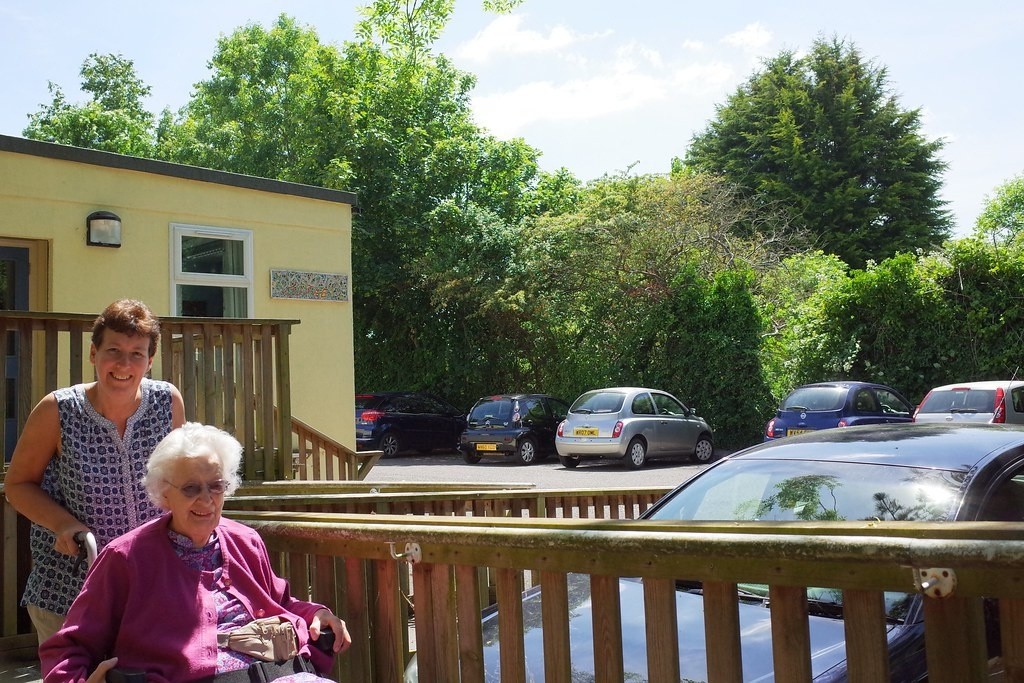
[218,615,298,662]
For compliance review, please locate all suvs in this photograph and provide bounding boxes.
[355,390,467,458]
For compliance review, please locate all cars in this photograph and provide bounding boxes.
[555,385,716,470]
[764,380,916,443]
[461,393,572,465]
[400,423,1024,683]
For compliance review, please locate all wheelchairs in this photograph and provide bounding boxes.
[72,530,345,683]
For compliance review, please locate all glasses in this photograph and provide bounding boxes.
[163,477,231,497]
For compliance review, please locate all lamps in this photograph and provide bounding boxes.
[87,211,123,248]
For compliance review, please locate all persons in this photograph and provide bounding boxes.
[5,299,186,682]
[37,422,352,682]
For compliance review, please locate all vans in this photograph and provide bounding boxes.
[912,379,1024,425]
